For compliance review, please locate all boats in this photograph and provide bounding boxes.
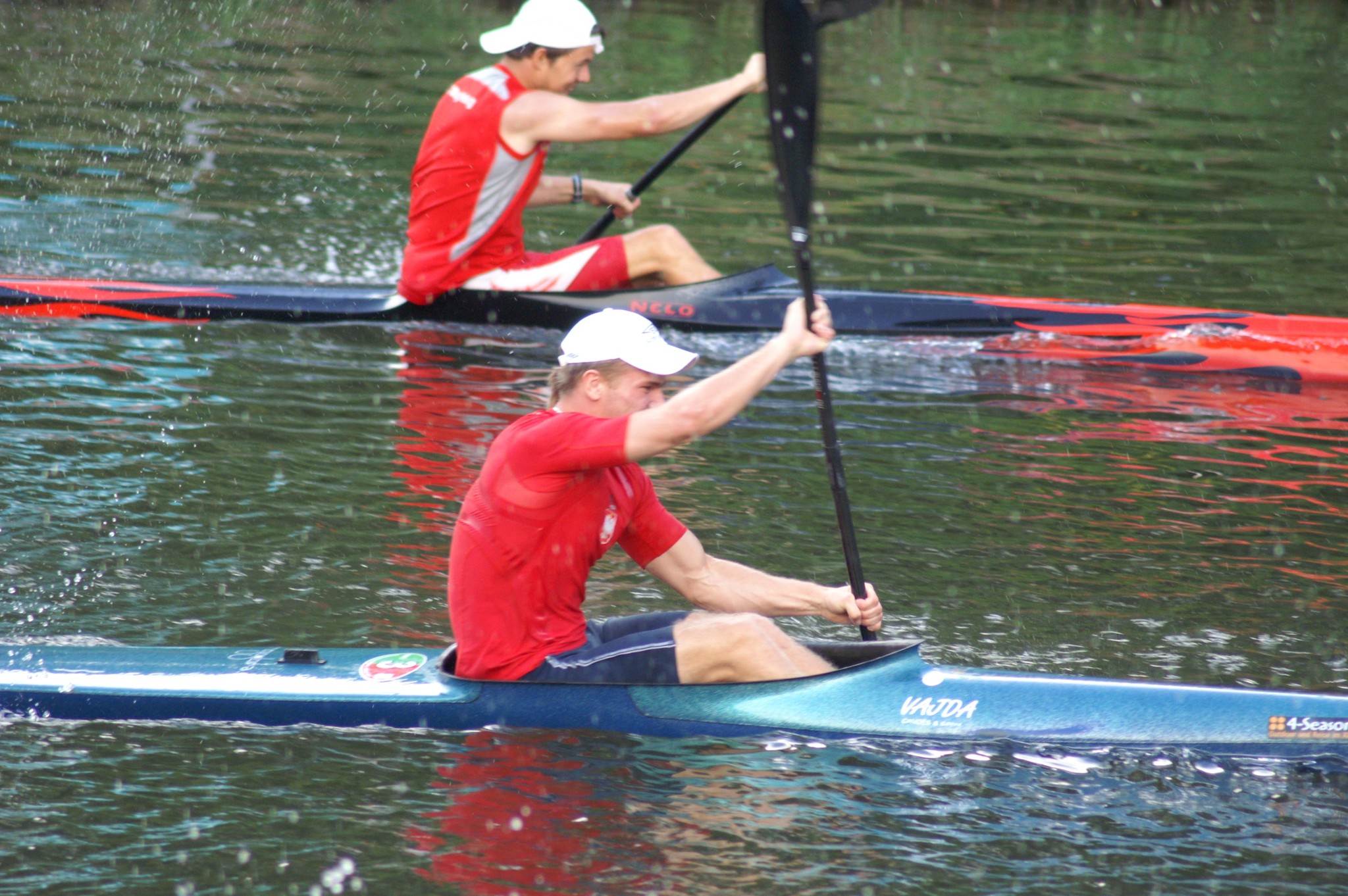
[0,267,1348,384]
[0,635,1348,763]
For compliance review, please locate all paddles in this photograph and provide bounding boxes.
[759,0,879,647]
[577,0,884,244]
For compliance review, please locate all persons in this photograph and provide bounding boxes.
[448,293,882,689]
[397,0,769,305]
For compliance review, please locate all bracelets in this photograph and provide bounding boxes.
[571,172,584,205]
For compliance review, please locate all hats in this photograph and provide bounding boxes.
[479,0,605,54]
[557,307,700,376]
